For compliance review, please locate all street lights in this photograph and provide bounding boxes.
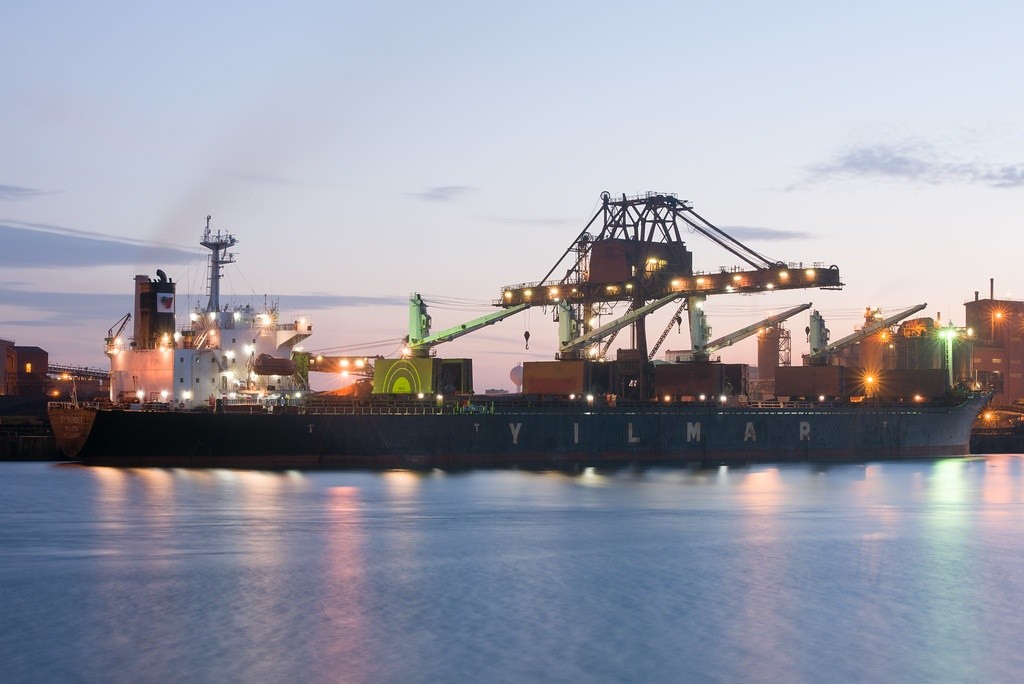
[61,375,77,407]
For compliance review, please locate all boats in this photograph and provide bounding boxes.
[46,215,995,465]
